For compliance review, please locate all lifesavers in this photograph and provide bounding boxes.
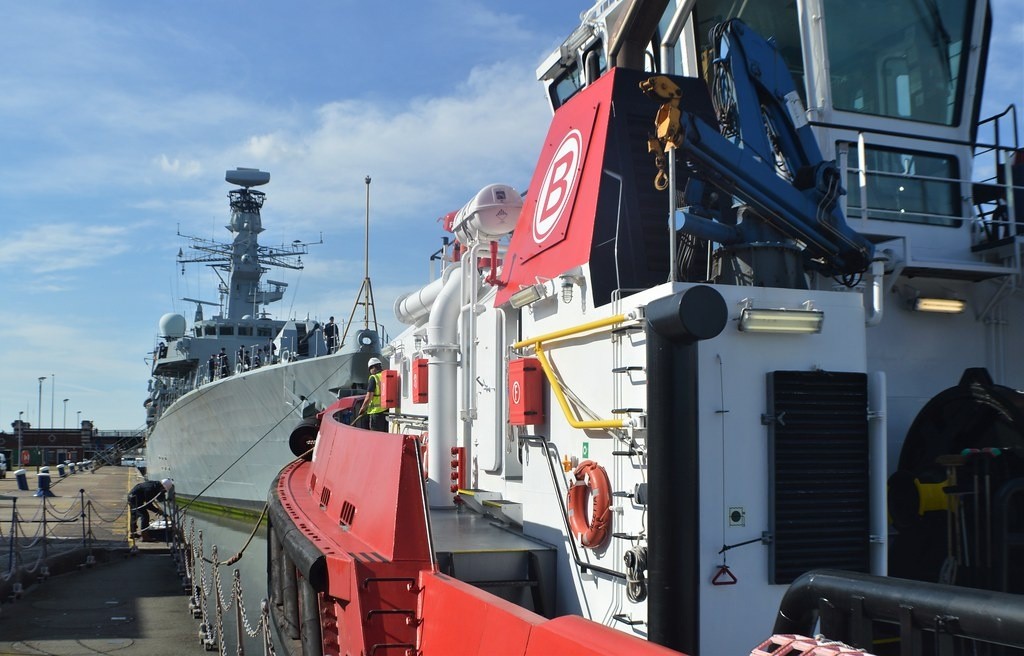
[22,450,29,464]
[566,459,612,548]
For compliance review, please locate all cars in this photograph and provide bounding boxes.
[0,453,7,478]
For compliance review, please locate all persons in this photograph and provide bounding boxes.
[208,354,216,383]
[265,338,277,362]
[237,345,262,371]
[323,316,339,355]
[359,357,389,433]
[217,347,230,377]
[127,478,174,539]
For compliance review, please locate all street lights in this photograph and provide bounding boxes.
[63,399,69,429]
[37,377,47,474]
[76,411,81,428]
[18,411,23,469]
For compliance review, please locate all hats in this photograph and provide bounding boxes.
[329,316,334,320]
[210,337,273,358]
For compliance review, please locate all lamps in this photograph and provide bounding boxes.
[733,298,825,335]
[911,298,968,315]
[510,286,545,309]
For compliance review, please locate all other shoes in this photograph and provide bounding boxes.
[130,533,142,539]
[142,536,156,542]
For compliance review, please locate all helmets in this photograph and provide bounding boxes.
[161,479,172,491]
[368,357,382,371]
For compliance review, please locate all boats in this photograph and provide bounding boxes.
[141,0,1024,656]
